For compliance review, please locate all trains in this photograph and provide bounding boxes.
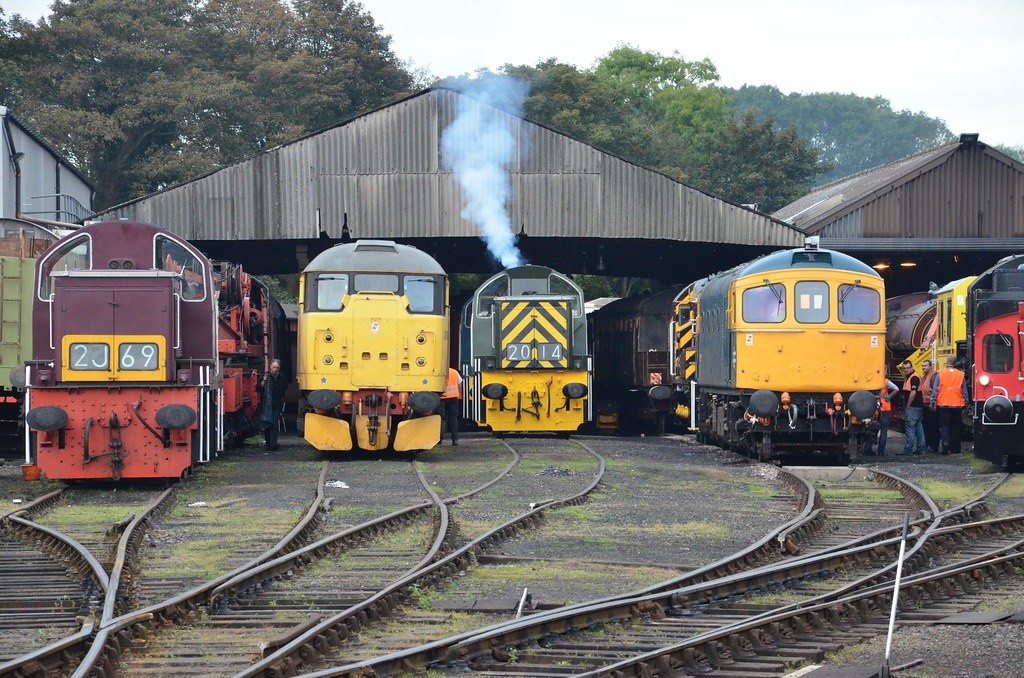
[451,264,593,439]
[294,239,451,457]
[876,253,1024,461]
[586,235,887,465]
[24,219,290,484]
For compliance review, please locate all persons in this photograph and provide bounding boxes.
[255,359,288,451]
[867,355,969,457]
[436,368,463,446]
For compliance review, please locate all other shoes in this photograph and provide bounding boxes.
[878,452,885,457]
[941,442,949,455]
[950,449,961,453]
[265,443,272,452]
[926,448,940,453]
[913,451,927,455]
[437,440,444,446]
[452,440,460,446]
[895,451,914,457]
[864,449,876,457]
[273,444,280,451]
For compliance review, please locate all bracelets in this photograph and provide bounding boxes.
[906,406,910,409]
[263,379,267,381]
